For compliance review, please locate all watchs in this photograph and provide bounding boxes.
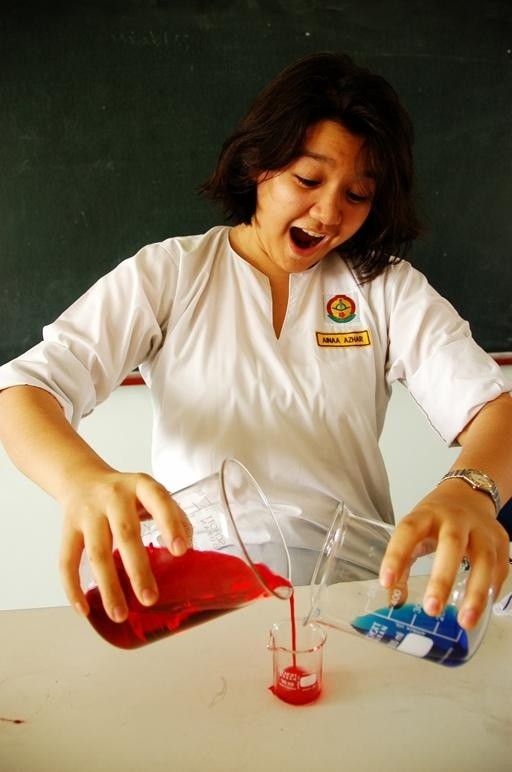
[437,468,501,516]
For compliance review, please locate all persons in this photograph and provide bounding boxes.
[0,49,512,635]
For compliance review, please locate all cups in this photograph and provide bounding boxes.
[270,621,326,706]
[79,458,297,651]
[303,501,493,668]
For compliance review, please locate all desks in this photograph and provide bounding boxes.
[1,573,512,771]
